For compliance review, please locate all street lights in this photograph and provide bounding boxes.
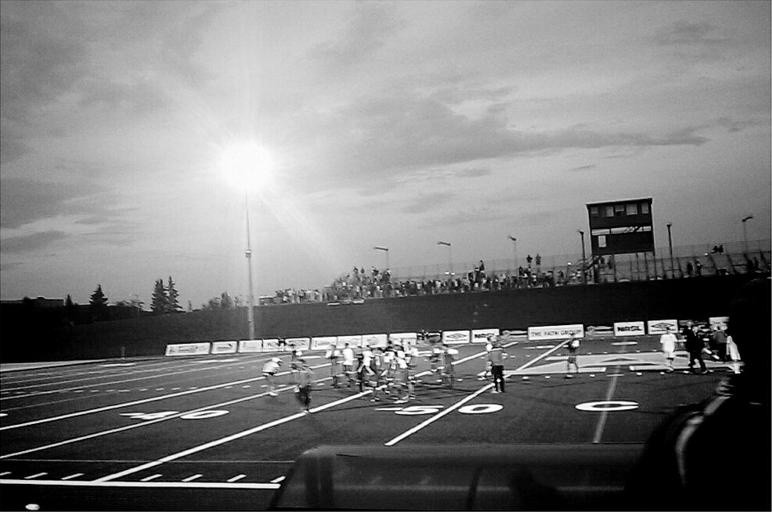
[569,227,589,286]
[224,143,280,345]
[502,233,521,289]
[739,214,758,276]
[663,219,678,281]
[370,246,393,299]
[434,240,455,295]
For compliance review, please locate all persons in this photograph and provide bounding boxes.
[680,319,742,375]
[261,336,504,414]
[660,327,676,373]
[567,332,578,377]
